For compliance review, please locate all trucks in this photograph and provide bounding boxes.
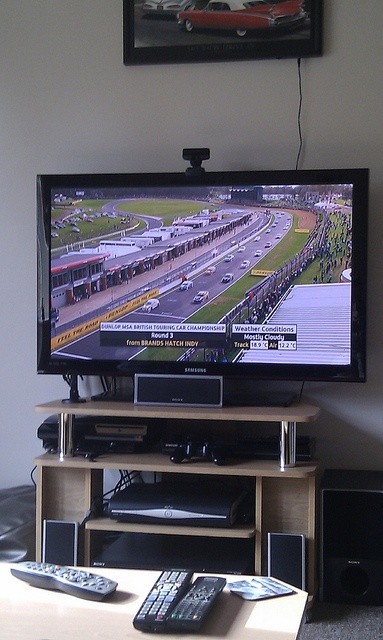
[63,209,220,258]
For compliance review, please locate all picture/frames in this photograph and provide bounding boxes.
[122,0,324,64]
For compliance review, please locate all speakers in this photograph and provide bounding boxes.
[318,468,383,605]
[42,520,81,567]
[267,532,312,624]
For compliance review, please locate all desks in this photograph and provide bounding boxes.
[0,563,308,640]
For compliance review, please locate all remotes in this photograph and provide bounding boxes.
[132,567,194,632]
[10,559,117,602]
[167,575,228,635]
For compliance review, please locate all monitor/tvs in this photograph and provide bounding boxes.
[34,169,370,410]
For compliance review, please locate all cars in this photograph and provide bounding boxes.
[275,233,281,239]
[50,211,106,233]
[221,272,233,283]
[254,249,262,257]
[252,236,261,242]
[192,290,209,303]
[179,281,193,291]
[223,254,234,263]
[265,229,271,233]
[240,260,250,268]
[271,211,291,230]
[204,265,215,275]
[141,298,160,312]
[237,246,246,252]
[142,0,195,17]
[264,242,272,248]
[176,0,308,37]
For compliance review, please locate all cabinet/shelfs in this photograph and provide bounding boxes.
[31,399,325,622]
